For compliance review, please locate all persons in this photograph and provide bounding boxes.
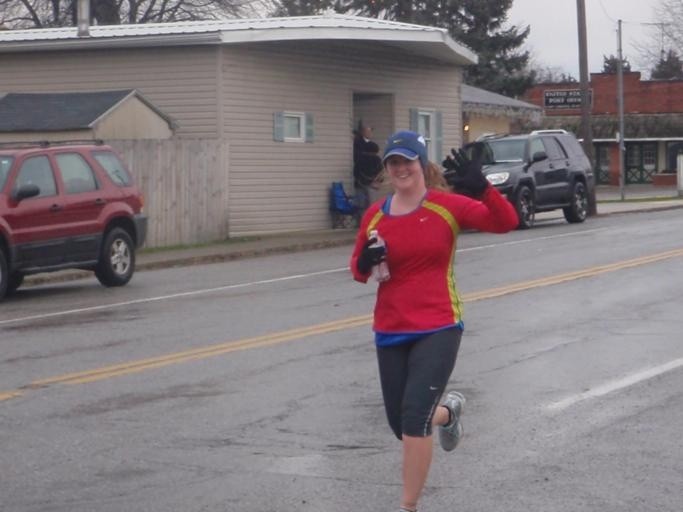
[354,119,384,227]
[349,129,519,512]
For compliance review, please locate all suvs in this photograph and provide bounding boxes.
[436,124,596,233]
[0,136,149,298]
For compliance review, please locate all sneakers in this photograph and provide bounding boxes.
[438,391,465,451]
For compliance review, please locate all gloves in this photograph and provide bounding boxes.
[445,147,487,192]
[357,237,387,274]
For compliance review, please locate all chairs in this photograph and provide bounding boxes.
[327,182,366,229]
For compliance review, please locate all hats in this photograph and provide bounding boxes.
[382,130,426,162]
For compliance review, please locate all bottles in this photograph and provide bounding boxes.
[369,229,392,284]
